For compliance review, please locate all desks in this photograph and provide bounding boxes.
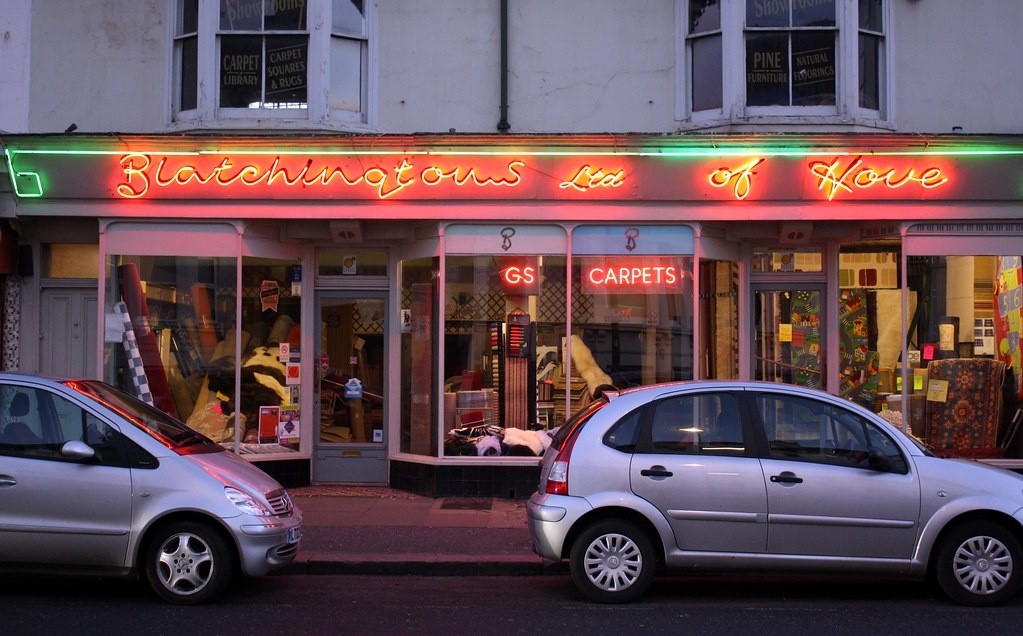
[538,406,554,430]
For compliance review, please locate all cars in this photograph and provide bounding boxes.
[526,379,1023,608]
[0,372,304,604]
